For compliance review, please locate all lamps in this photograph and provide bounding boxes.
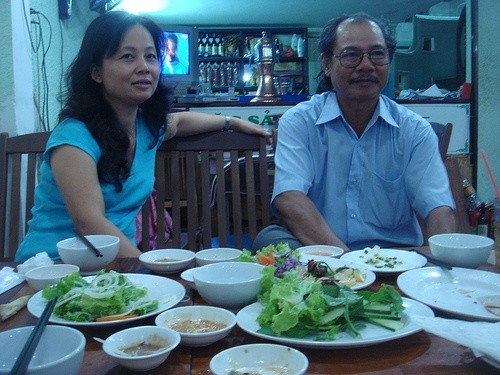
[250,31,282,103]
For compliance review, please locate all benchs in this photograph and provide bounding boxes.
[0,132,272,264]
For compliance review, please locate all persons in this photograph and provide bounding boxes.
[13,11,272,263]
[161,34,188,74]
[253,13,457,251]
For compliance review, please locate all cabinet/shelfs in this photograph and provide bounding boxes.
[199,28,309,95]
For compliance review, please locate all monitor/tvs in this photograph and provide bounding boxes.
[154,24,199,94]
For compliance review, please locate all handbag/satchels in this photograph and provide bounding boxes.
[135,187,175,252]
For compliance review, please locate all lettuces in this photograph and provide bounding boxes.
[40,268,159,323]
[237,242,406,341]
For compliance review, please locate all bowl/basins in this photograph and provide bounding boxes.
[56,235,120,275]
[0,324,88,375]
[429,233,495,270]
[103,326,181,371]
[138,248,196,273]
[195,247,243,264]
[25,263,80,291]
[156,306,236,346]
[209,343,309,375]
[181,267,200,289]
[295,245,343,257]
[192,261,267,306]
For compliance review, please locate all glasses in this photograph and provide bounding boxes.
[332,45,394,67]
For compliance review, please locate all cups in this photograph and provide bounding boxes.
[228,87,235,96]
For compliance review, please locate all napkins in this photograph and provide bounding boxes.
[17,251,54,274]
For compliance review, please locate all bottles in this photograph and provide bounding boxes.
[198,33,225,58]
[199,62,239,88]
[462,176,482,210]
[476,202,489,237]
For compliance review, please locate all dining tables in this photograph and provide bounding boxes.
[0,257,500,375]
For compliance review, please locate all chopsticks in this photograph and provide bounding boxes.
[412,248,455,271]
[10,296,58,375]
[74,229,105,258]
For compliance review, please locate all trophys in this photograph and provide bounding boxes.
[251,30,285,102]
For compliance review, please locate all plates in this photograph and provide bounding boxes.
[341,247,428,278]
[27,272,186,325]
[396,264,500,321]
[236,293,435,350]
[278,254,376,290]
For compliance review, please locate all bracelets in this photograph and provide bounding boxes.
[223,114,232,130]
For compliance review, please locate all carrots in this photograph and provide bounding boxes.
[257,246,275,265]
[95,312,139,322]
